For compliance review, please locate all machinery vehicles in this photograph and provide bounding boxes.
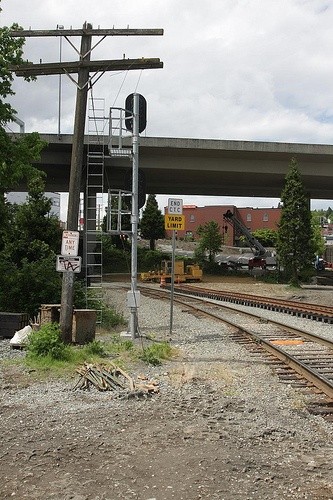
[222,210,269,270]
[141,260,204,282]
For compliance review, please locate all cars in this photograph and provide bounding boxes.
[312,259,332,268]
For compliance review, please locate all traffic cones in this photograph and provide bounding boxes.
[160,276,165,287]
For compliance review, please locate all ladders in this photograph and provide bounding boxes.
[84,119,105,325]
